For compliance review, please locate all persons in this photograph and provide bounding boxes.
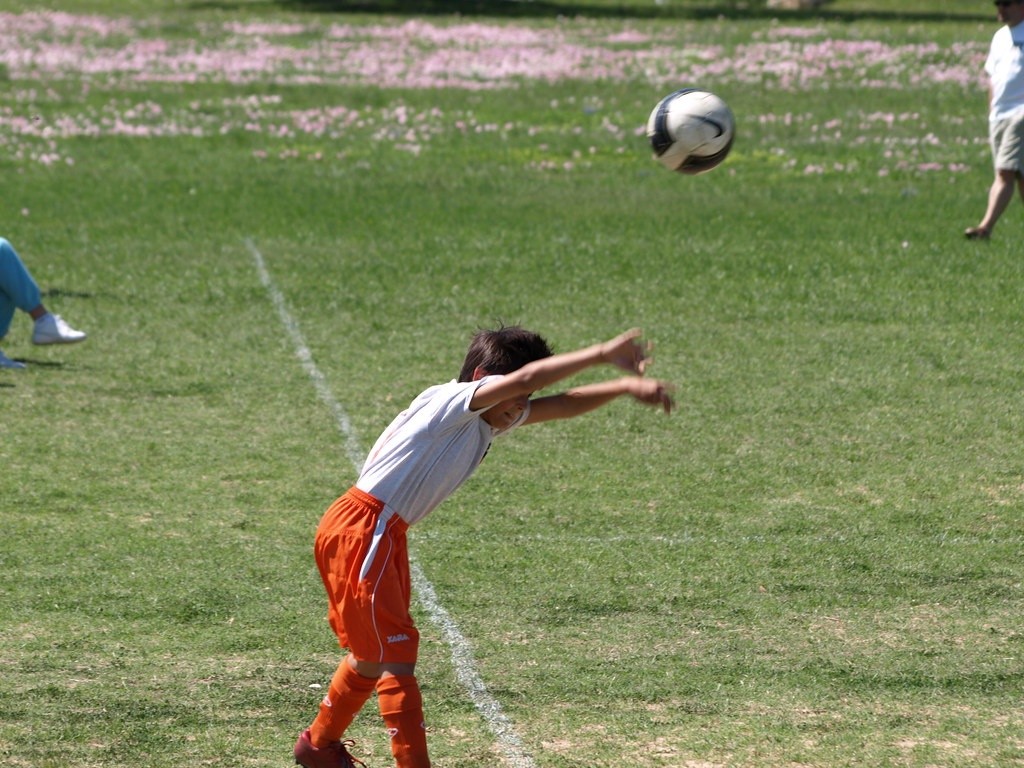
[0,236,88,369]
[963,0,1024,241]
[293,314,678,768]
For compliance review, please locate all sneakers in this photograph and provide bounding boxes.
[31,314,86,345]
[294,726,369,768]
[0,350,25,369]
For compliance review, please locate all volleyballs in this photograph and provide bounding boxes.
[646,88,737,176]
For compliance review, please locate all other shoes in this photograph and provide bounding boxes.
[965,227,990,241]
